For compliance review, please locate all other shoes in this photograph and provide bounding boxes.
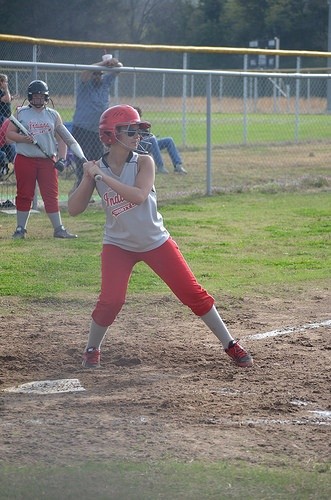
[159,167,172,176]
[173,165,188,174]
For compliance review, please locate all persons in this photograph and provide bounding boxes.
[66,105,253,371]
[0,57,187,238]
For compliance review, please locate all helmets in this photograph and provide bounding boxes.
[27,80,49,102]
[98,104,152,145]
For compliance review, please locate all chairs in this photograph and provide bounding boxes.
[62,122,78,180]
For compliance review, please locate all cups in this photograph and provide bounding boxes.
[102,54,112,66]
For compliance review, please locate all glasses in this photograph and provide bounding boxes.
[122,128,146,138]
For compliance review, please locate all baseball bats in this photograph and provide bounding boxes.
[56,124,102,182]
[8,114,57,164]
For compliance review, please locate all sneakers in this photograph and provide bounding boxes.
[12,224,27,239]
[224,339,253,367]
[53,224,78,238]
[81,347,101,367]
